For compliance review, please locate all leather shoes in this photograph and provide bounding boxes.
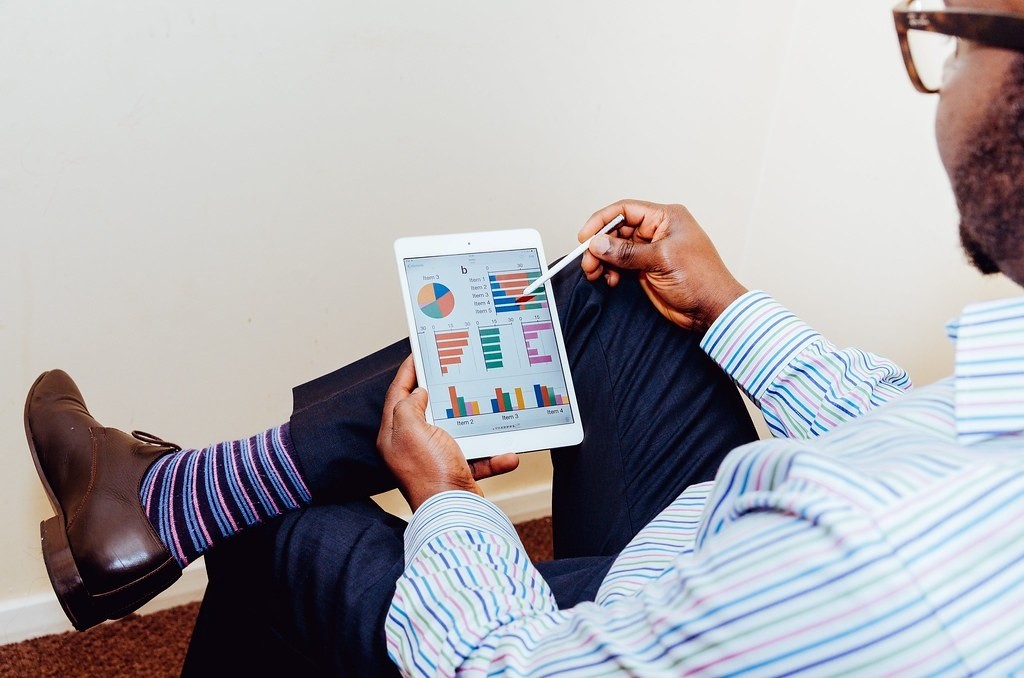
[23,369,183,632]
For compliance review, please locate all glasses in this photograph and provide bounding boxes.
[893,0,1024,93]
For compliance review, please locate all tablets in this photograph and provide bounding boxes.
[393,227,585,460]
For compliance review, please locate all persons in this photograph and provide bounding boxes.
[23,1,1023,678]
